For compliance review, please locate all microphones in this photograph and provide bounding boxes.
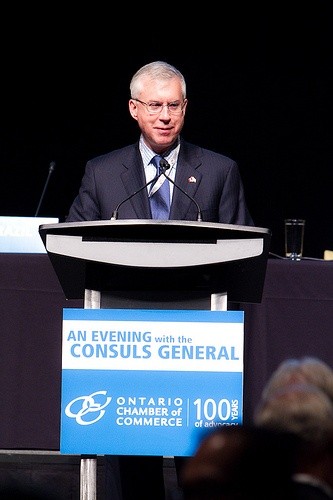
[111,159,202,222]
[35,161,57,216]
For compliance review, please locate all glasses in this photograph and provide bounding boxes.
[132,98,185,113]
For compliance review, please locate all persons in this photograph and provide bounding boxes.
[181,357,333,500]
[65,61,256,227]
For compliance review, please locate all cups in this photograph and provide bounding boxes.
[284,219,304,262]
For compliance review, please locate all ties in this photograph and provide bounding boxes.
[148,155,170,220]
